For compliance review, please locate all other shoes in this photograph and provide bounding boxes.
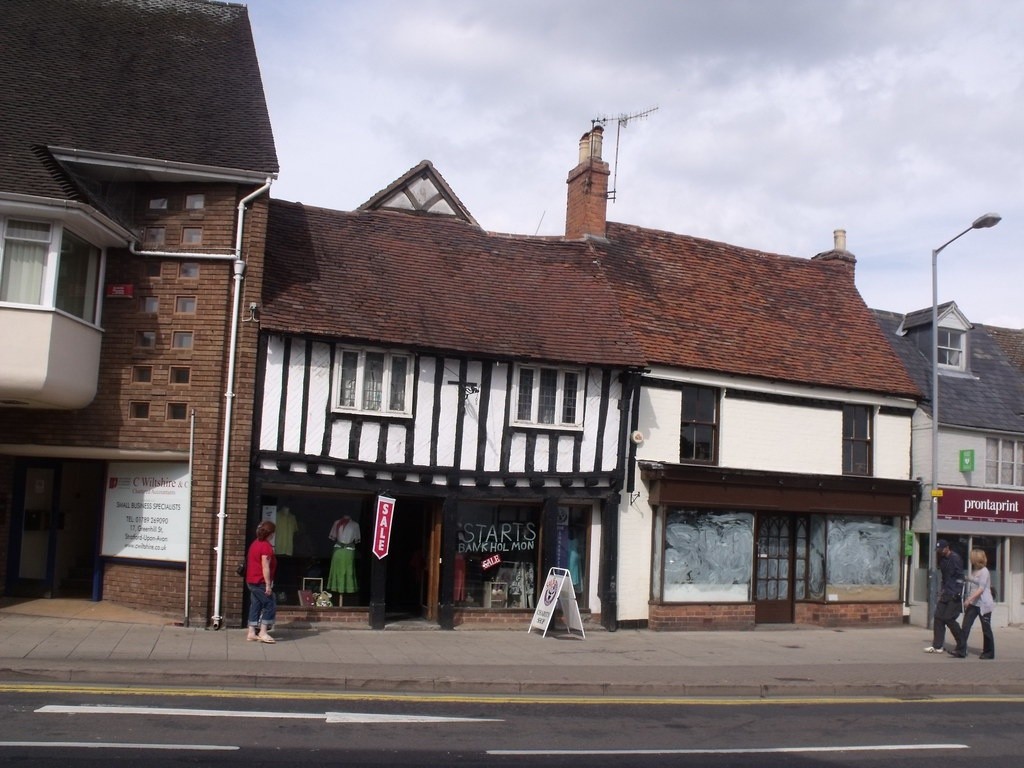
[924,646,944,653]
[979,655,995,659]
[947,649,965,658]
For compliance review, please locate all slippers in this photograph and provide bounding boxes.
[257,633,276,644]
[247,634,260,642]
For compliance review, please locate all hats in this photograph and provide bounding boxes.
[935,539,949,552]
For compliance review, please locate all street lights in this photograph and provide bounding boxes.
[927,211,1002,629]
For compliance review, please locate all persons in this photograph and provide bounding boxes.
[327,515,360,592]
[924,539,995,659]
[246,521,276,642]
